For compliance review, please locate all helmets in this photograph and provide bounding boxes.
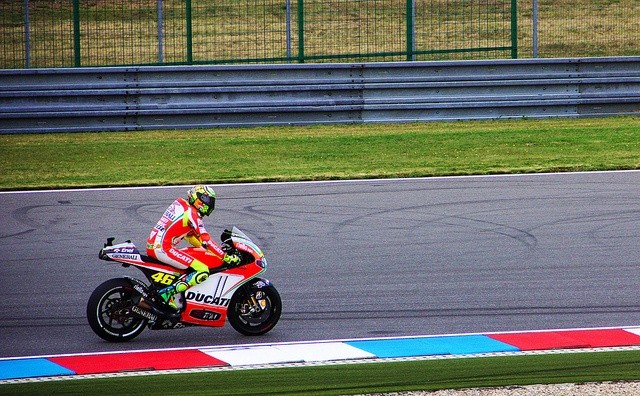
[187,185,216,217]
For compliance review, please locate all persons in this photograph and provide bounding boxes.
[145,185,241,313]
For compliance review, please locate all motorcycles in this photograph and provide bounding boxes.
[86,226,282,343]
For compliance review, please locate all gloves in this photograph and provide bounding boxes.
[224,254,241,266]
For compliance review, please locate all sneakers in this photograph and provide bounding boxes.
[157,285,179,314]
